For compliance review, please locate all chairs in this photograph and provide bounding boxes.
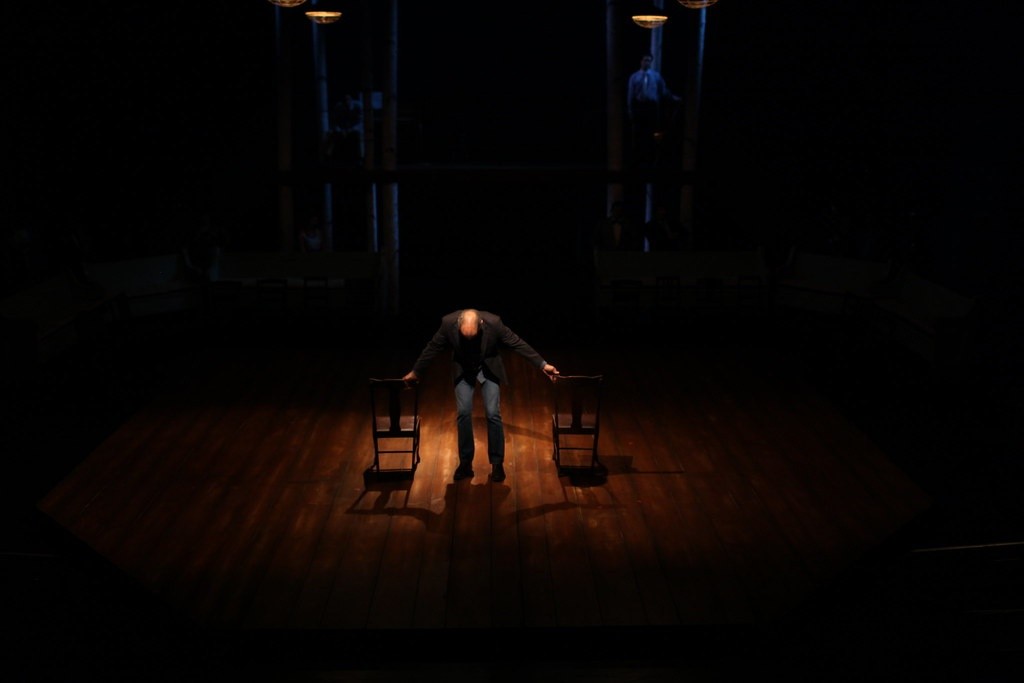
[369,378,421,479]
[551,373,603,477]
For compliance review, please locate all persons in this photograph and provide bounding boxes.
[627,53,683,138]
[587,201,644,253]
[398,310,559,484]
[318,94,367,159]
[3,193,237,295]
[295,210,330,252]
